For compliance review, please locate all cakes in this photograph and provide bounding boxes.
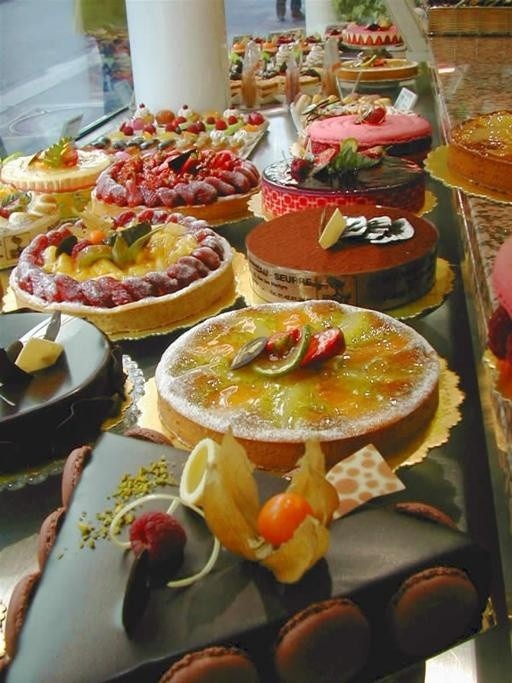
[343,20,405,45]
[299,108,431,164]
[11,209,234,337]
[0,310,131,474]
[446,110,512,195]
[337,58,419,80]
[243,205,439,308]
[0,148,111,221]
[226,31,328,103]
[84,101,264,154]
[0,187,61,270]
[261,140,424,217]
[299,93,392,154]
[0,428,499,683]
[91,150,260,227]
[155,298,441,473]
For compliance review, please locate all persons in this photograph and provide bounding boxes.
[274,0,306,24]
